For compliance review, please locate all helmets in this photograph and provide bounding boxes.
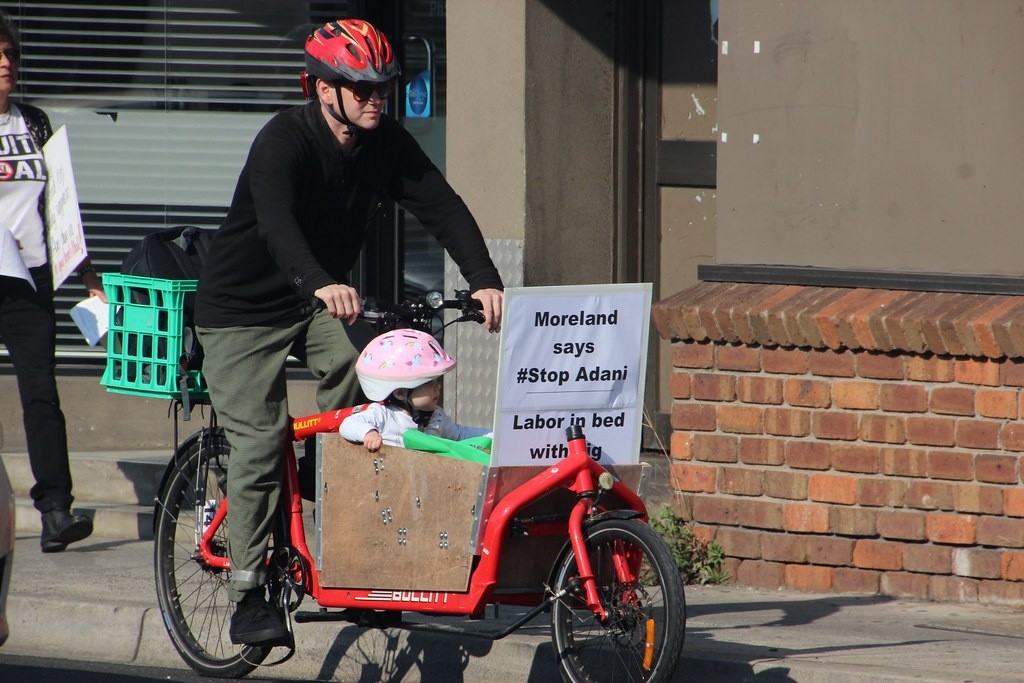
[302,17,404,88]
[355,326,456,404]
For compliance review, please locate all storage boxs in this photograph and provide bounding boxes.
[100,273,210,399]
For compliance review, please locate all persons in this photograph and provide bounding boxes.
[190,20,504,645]
[0,11,109,555]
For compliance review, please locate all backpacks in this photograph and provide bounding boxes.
[122,224,215,287]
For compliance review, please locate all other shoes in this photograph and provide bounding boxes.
[228,587,295,649]
[39,508,93,552]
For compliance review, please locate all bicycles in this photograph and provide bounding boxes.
[147,288,684,683]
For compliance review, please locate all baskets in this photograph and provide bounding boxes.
[94,269,198,400]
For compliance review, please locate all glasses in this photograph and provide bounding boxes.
[349,80,395,100]
